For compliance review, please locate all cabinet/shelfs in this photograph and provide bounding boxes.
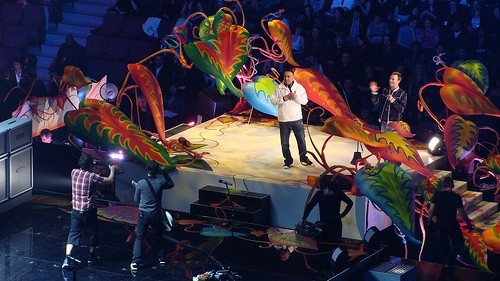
[0,118,34,204]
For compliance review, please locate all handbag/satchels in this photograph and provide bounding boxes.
[162,211,174,232]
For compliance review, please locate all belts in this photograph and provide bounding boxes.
[382,121,392,125]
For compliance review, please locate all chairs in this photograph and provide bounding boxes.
[0,2,193,117]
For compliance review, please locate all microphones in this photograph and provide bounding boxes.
[67,255,82,264]
[219,180,233,185]
[288,84,292,93]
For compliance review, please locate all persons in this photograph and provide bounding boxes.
[296,175,354,258]
[427,177,476,276]
[369,71,408,168]
[113,0,500,134]
[270,70,315,169]
[55,33,92,84]
[61,153,116,269]
[130,160,175,271]
[5,60,30,116]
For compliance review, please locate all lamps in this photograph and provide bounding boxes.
[426,134,445,156]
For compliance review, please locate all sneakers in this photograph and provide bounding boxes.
[88,256,100,263]
[283,158,294,168]
[456,254,477,268]
[130,263,137,270]
[62,258,70,268]
[159,258,165,263]
[301,156,315,166]
